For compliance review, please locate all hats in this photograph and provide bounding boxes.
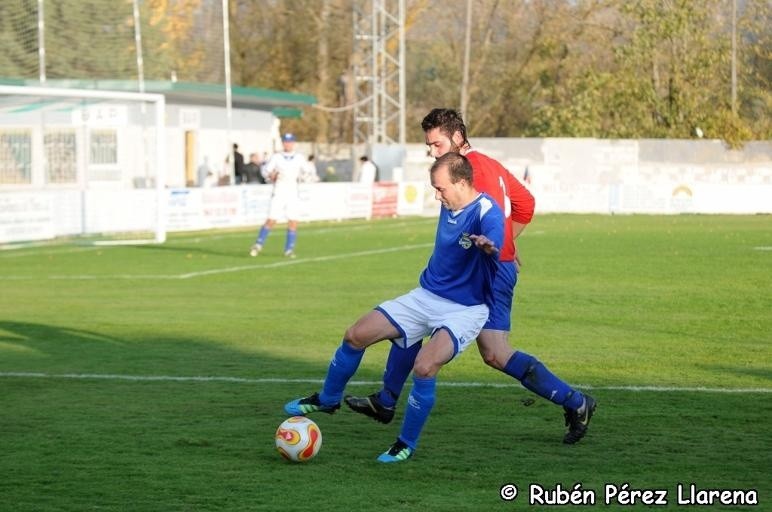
[283,133,295,141]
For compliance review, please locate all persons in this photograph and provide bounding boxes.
[250,133,304,259]
[226,144,321,185]
[357,157,376,181]
[343,109,597,444]
[284,153,504,463]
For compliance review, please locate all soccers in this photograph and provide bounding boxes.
[275,416,322,464]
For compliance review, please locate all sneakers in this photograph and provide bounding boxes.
[285,393,342,417]
[377,441,412,463]
[344,394,395,424]
[563,395,597,444]
